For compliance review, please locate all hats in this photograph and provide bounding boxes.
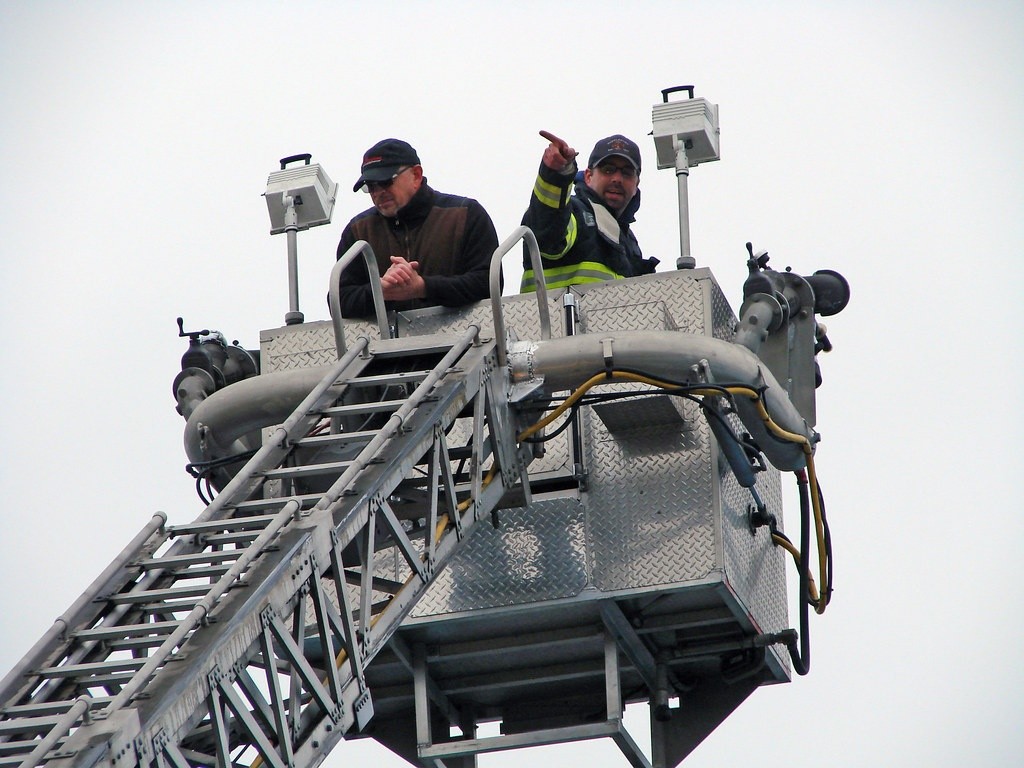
[352,138,421,192]
[588,134,641,171]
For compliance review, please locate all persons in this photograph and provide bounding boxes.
[326,138,504,315]
[520,129,657,294]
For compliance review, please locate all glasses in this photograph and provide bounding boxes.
[596,164,639,176]
[360,165,413,193]
